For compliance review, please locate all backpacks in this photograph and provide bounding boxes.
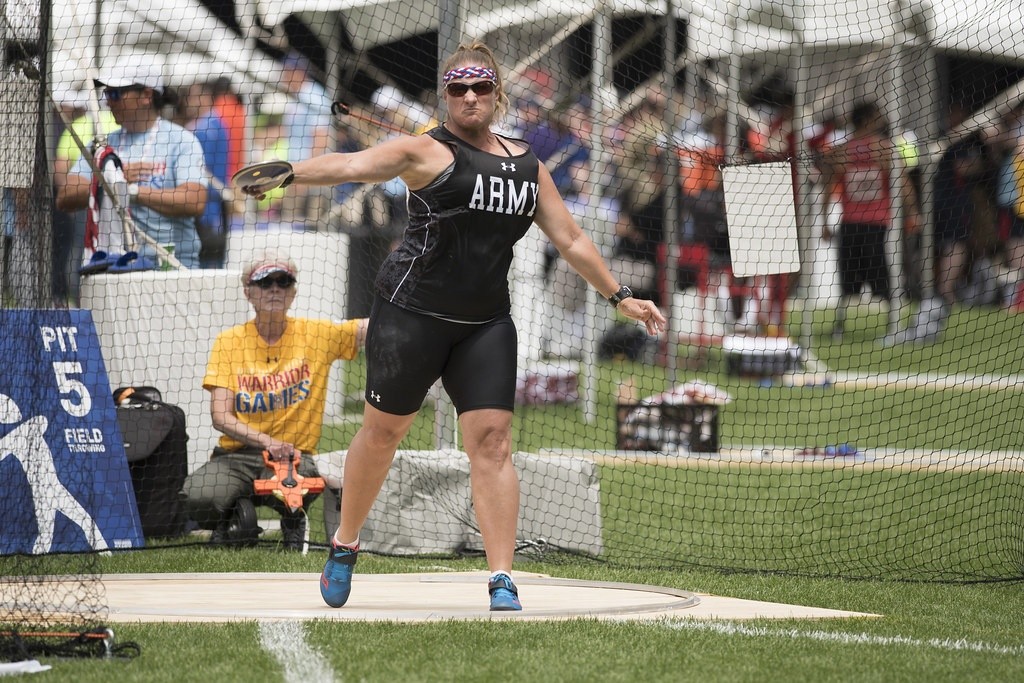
[110,385,188,535]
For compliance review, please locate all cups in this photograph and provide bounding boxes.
[157,244,176,270]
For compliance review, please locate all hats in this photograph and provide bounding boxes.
[93,60,165,95]
[244,263,297,286]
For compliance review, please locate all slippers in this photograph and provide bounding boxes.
[107,251,153,274]
[79,251,123,275]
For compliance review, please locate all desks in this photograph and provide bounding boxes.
[79,269,250,475]
[224,230,351,422]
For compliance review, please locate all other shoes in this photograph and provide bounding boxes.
[279,512,310,557]
[209,523,228,547]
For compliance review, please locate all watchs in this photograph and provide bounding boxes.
[607,285,633,308]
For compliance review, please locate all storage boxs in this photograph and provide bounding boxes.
[616,404,719,454]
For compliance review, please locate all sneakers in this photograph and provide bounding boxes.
[490,573,522,612]
[320,541,359,608]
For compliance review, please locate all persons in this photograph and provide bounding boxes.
[259,48,424,254]
[504,74,801,337]
[54,52,212,267]
[178,253,375,555]
[805,98,920,352]
[918,98,1022,350]
[3,71,111,307]
[242,39,664,612]
[157,51,247,269]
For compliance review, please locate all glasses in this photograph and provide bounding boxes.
[447,81,496,97]
[102,84,143,100]
[254,276,293,289]
